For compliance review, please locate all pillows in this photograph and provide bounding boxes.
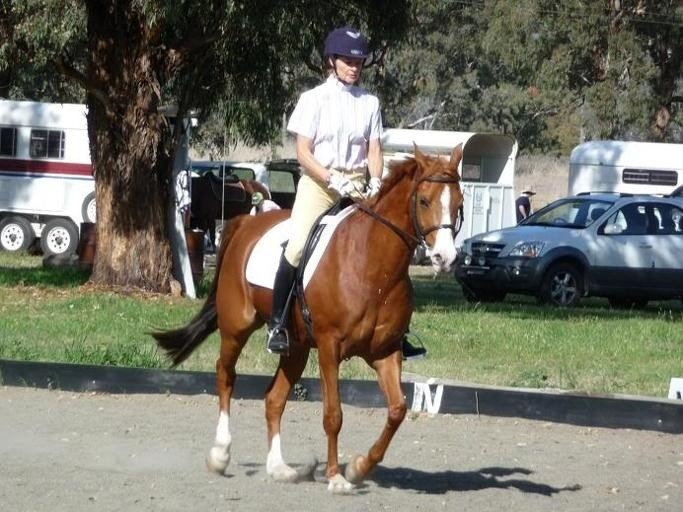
[323,27,369,58]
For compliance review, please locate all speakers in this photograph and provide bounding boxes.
[402,336,426,357]
[267,248,301,351]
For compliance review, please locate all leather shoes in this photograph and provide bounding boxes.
[327,167,356,198]
[366,176,382,199]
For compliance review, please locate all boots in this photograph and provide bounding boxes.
[453,188,682,309]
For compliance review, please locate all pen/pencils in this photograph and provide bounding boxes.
[186,157,305,211]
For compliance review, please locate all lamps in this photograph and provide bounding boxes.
[589,207,682,233]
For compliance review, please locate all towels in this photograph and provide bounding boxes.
[80,223,97,270]
[185,229,204,287]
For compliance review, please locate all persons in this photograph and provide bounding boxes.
[267,27,426,357]
[249,191,281,216]
[516,186,536,223]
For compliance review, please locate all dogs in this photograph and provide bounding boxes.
[190,175,269,252]
[141,140,467,495]
